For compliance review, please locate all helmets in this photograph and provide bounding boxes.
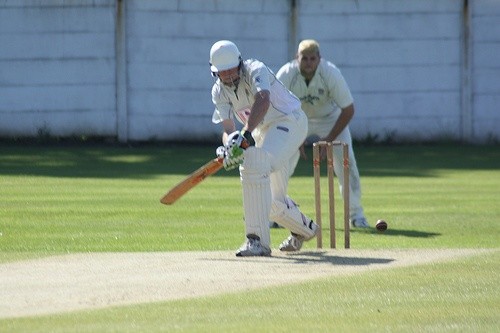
[210,40,245,79]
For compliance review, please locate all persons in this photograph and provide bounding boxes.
[267,39,370,229]
[209,40,321,257]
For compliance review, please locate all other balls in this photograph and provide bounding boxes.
[376,221,387,232]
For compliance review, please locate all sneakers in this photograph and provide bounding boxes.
[278,220,321,251]
[352,218,370,228]
[236,240,272,256]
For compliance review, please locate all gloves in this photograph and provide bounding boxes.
[222,145,245,171]
[225,130,255,152]
